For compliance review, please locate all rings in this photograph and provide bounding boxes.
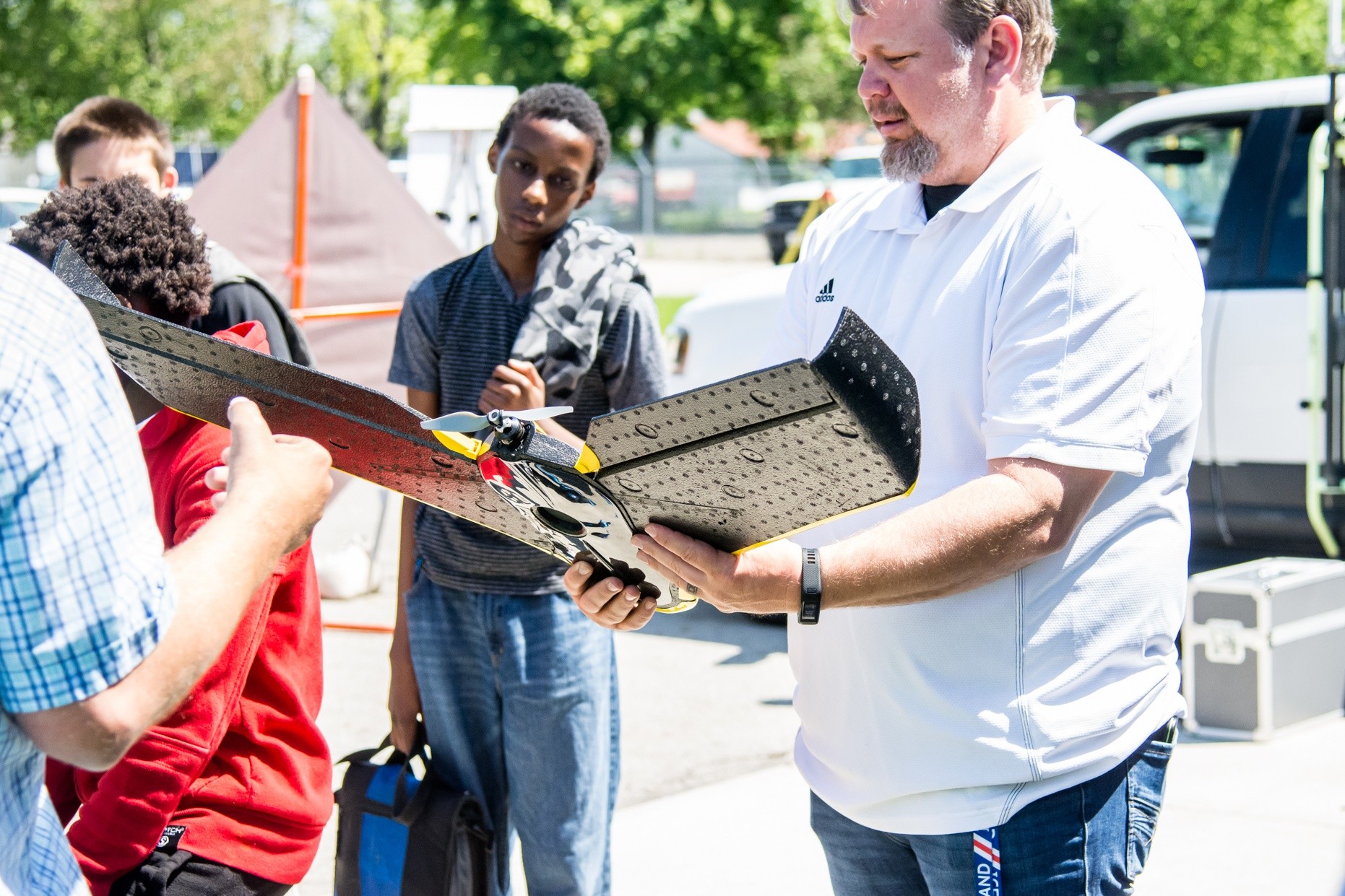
[687,583,698,595]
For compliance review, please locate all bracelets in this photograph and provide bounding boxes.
[797,548,823,625]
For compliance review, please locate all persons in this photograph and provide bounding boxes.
[388,81,666,896]
[563,0,1207,896]
[0,97,334,895]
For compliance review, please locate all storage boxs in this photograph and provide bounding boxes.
[1182,556,1345,738]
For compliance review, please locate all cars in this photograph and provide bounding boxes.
[0,187,53,232]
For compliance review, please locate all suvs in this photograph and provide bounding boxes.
[1083,73,1345,563]
[758,142,894,269]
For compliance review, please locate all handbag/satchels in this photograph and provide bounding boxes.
[333,719,496,896]
